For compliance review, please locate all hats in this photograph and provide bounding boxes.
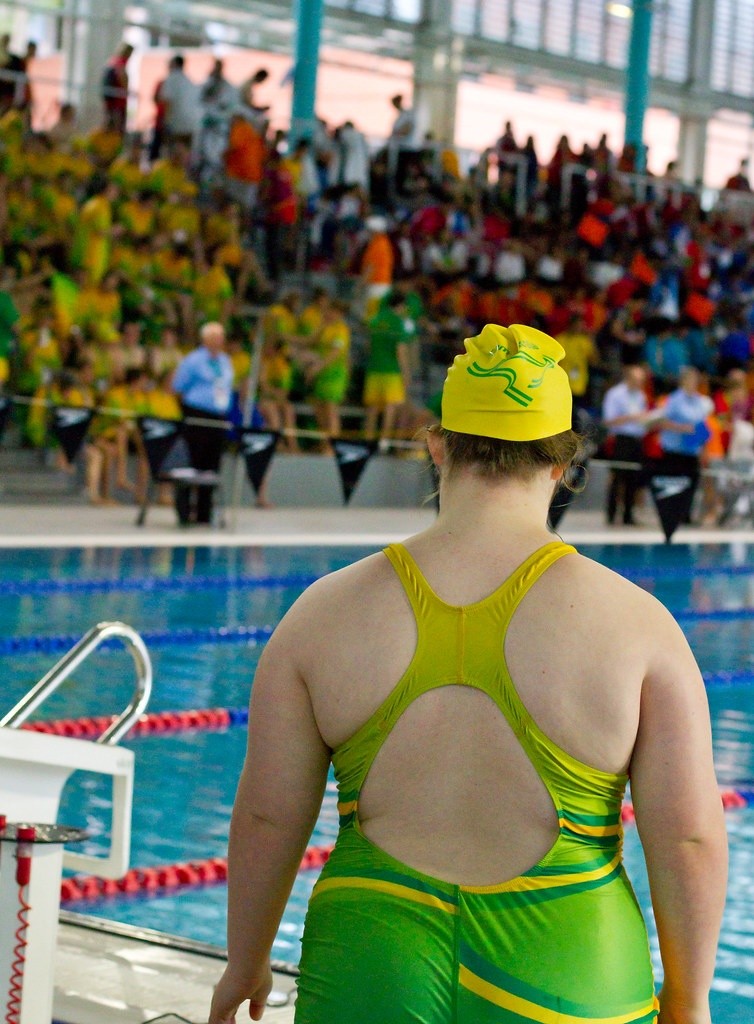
[365,216,388,232]
[442,324,572,441]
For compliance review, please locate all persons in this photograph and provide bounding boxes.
[206,324,725,1024]
[2,34,754,537]
